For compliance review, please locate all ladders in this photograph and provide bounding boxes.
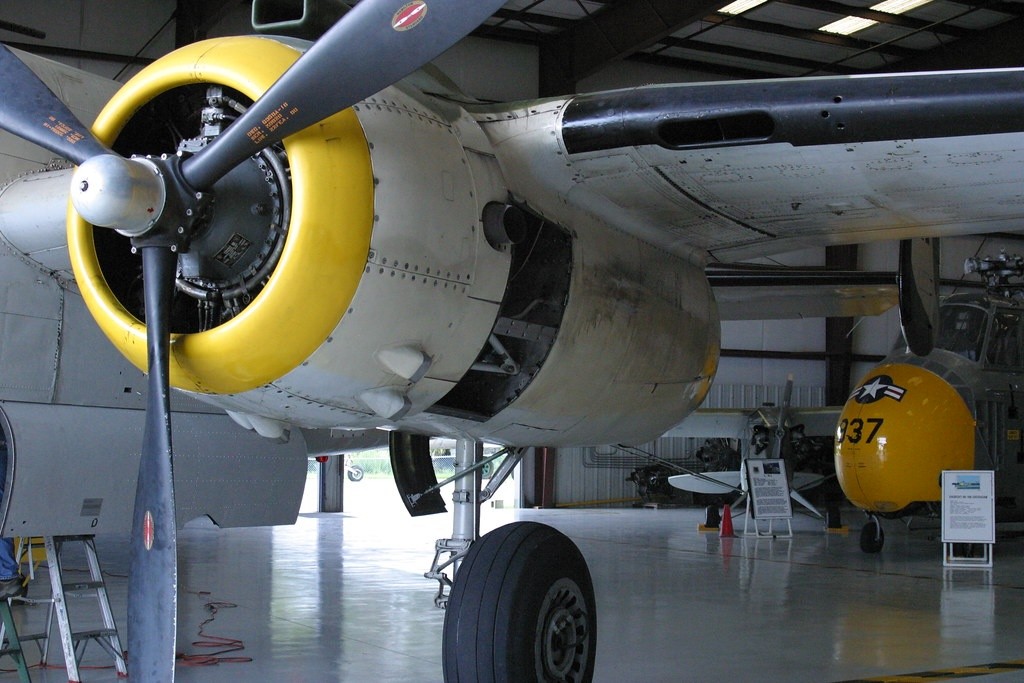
[0,536,129,682]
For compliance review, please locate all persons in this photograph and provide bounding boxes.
[0,536,29,605]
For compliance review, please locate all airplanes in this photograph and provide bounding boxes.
[0,1,1024,682]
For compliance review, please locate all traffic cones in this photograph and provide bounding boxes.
[718,536,732,573]
[717,503,739,539]
